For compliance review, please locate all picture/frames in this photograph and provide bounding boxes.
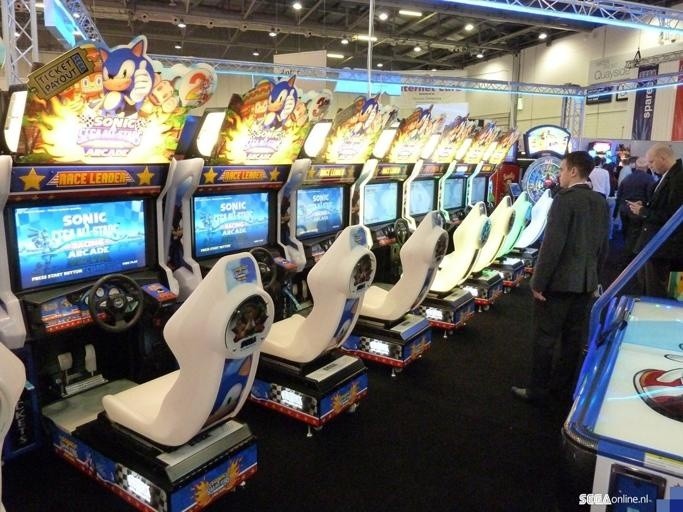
[586,79,628,105]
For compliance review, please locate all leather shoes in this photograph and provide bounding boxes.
[512,386,549,401]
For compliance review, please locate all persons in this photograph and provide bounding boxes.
[617,157,657,257]
[623,142,682,301]
[508,150,612,406]
[588,152,638,198]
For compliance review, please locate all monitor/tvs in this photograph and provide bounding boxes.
[191,188,273,261]
[409,178,437,218]
[364,180,399,227]
[296,182,350,240]
[443,177,466,211]
[4,194,155,296]
[471,175,487,206]
